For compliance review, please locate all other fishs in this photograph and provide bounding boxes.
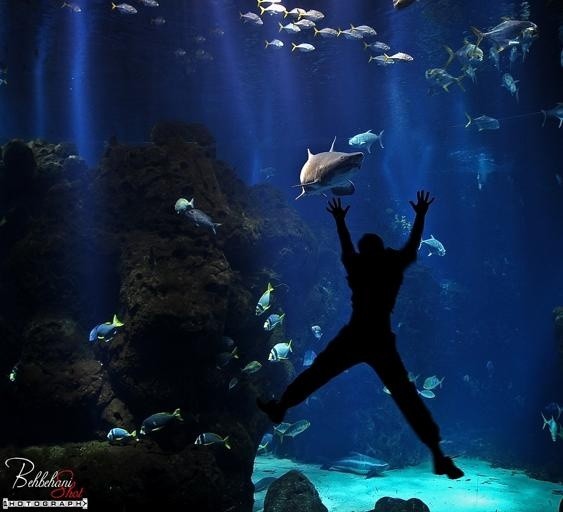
[110,0,540,96]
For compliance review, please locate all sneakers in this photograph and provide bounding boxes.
[256,394,286,425]
[432,455,463,479]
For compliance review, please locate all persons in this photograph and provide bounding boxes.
[253,189,465,480]
